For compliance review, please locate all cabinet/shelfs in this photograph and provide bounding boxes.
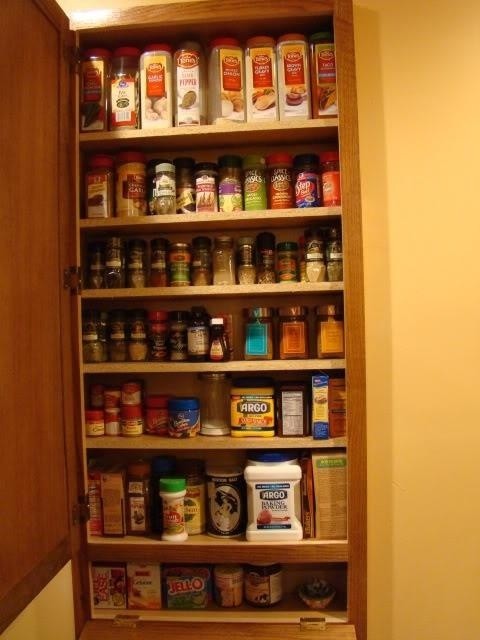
[0,0,369,639]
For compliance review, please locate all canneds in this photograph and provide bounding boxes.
[213,562,284,610]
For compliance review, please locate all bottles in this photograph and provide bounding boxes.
[197,371,232,438]
